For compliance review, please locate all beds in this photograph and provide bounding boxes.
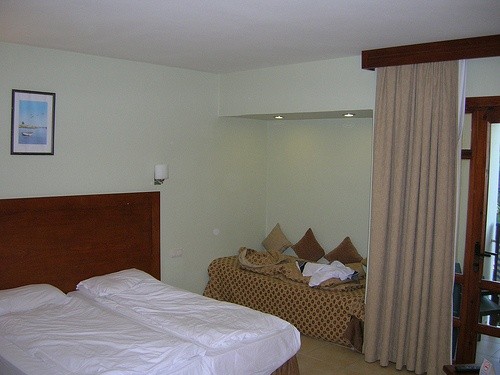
[0,191,301,375]
[200,254,368,351]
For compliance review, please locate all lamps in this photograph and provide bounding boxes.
[154,165,168,185]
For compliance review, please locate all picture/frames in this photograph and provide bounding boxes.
[11,89,56,155]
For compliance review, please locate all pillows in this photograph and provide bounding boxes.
[1,284,73,317]
[323,236,364,266]
[261,222,293,258]
[291,227,326,266]
[75,271,159,302]
